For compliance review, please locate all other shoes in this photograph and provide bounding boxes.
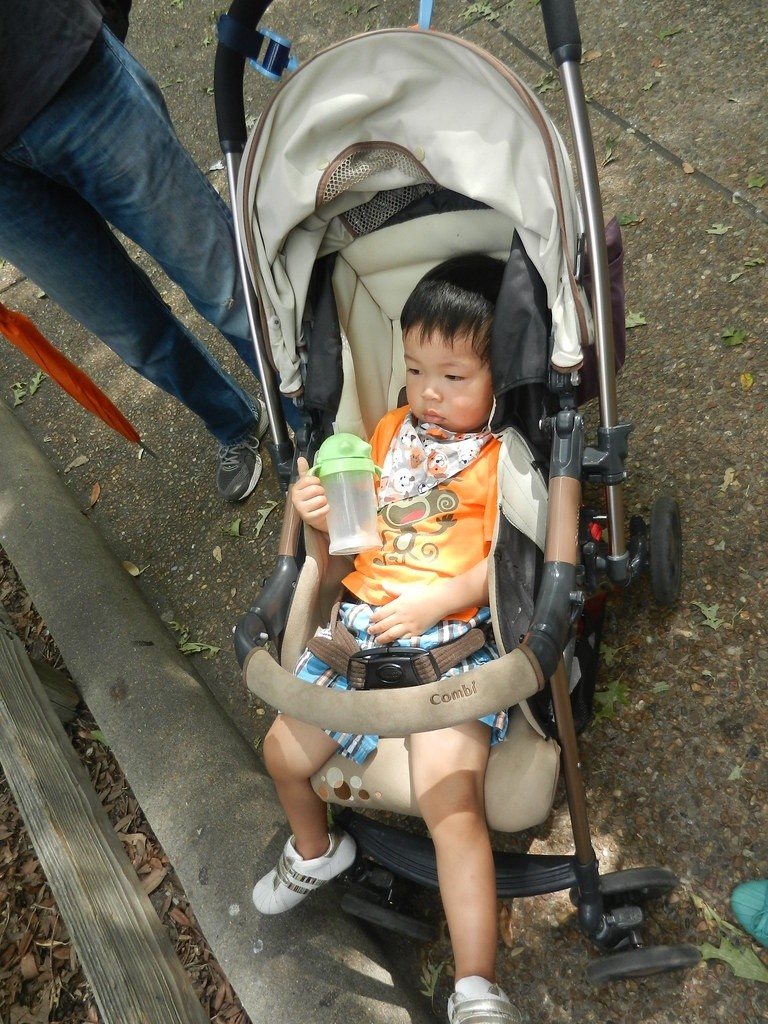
[731,880,768,948]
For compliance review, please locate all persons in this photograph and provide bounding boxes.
[251,250,531,1024]
[0,0,272,499]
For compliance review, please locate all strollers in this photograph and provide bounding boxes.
[212,0,700,989]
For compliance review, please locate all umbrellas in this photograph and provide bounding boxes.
[0,304,159,465]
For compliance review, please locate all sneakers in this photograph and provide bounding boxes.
[252,826,357,916]
[447,975,522,1024]
[215,397,270,502]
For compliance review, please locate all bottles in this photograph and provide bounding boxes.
[305,422,383,556]
